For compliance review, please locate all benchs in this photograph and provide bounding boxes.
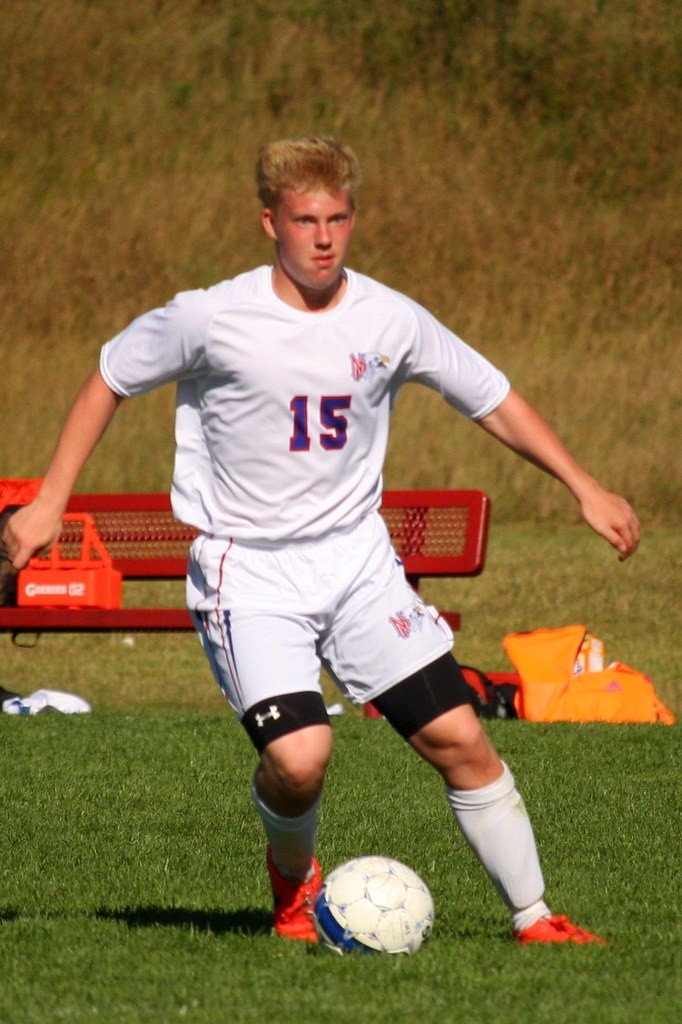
[0,489,492,717]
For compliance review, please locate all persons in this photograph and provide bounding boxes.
[2,132,646,939]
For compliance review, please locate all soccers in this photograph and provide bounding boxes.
[310,850,440,961]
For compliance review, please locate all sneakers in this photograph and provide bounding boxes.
[267,846,323,944]
[513,914,609,947]
